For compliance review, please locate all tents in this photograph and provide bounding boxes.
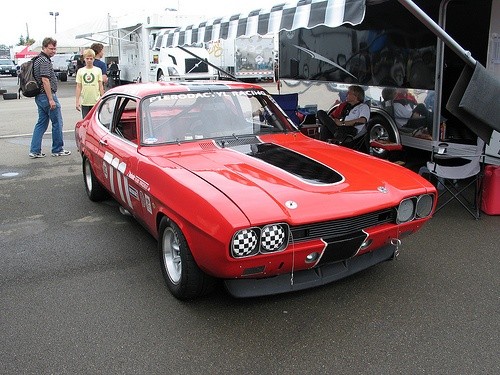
[16,45,40,58]
[28,36,108,52]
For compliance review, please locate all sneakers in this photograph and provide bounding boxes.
[52,149,71,157]
[29,152,45,158]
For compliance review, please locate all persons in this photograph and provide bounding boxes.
[29,37,70,159]
[76,49,104,119]
[317,85,370,142]
[91,43,117,86]
[383,88,432,136]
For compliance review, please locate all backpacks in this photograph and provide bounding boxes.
[16,55,49,97]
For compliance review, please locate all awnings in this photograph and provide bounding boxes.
[151,0,500,79]
[74,24,143,44]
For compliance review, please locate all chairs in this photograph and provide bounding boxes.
[299,114,316,125]
[427,136,486,220]
[259,92,308,126]
[200,97,245,132]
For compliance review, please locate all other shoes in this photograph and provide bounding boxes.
[334,127,342,139]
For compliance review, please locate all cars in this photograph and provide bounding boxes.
[73,79,439,304]
[51,54,69,85]
[0,57,19,76]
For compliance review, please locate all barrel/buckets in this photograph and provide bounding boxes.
[481,165,500,215]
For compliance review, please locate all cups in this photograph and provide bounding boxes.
[438,143,449,154]
